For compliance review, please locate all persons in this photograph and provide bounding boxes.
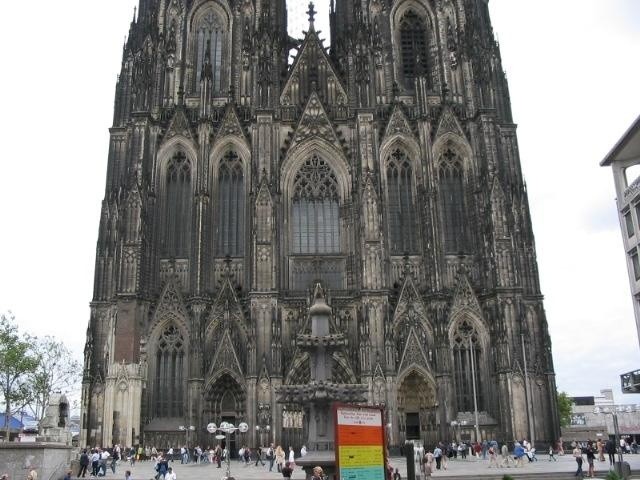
[311,465,329,480]
[422,436,640,471]
[76,441,308,480]
[393,467,401,480]
[424,458,433,480]
[26,470,38,480]
[62,470,73,480]
[586,443,596,478]
[0,472,9,480]
[573,444,584,477]
[387,457,393,480]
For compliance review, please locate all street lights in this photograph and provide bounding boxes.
[255,424,271,447]
[592,403,640,478]
[458,320,481,444]
[460,420,468,441]
[178,425,196,454]
[450,420,459,442]
[206,420,250,480]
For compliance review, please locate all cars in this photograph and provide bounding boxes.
[22,420,40,434]
